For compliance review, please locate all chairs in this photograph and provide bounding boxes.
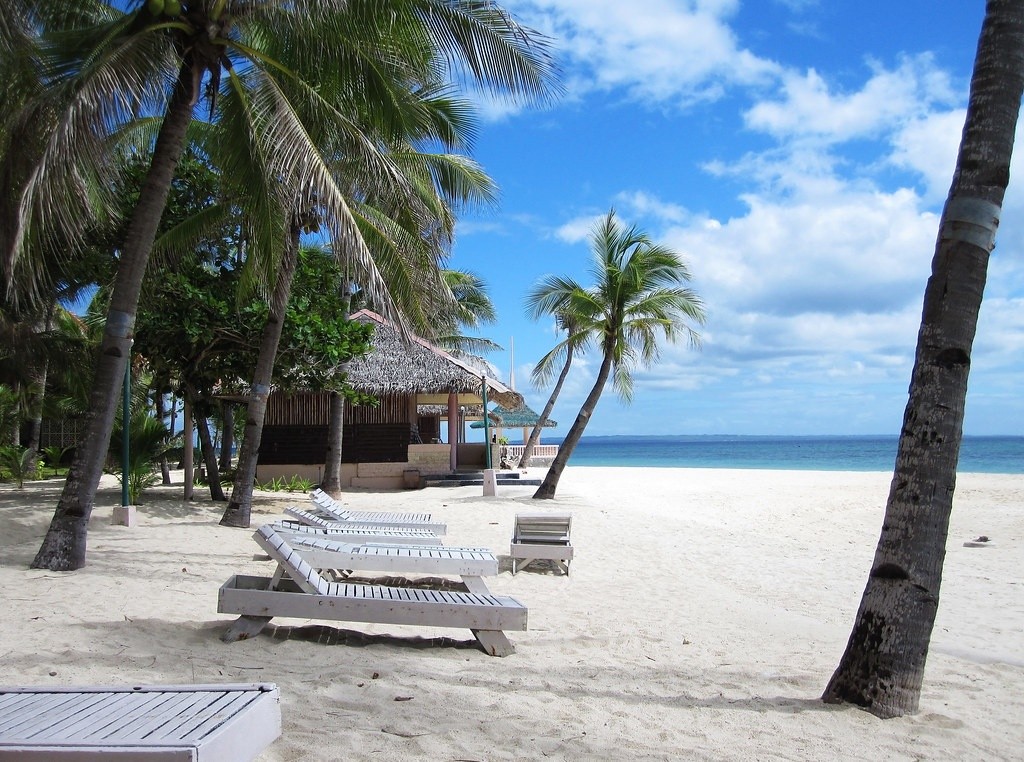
[272,520,441,545]
[509,513,574,577]
[309,488,431,523]
[311,498,447,535]
[282,505,438,535]
[271,524,500,595]
[0,680,284,762]
[217,524,528,658]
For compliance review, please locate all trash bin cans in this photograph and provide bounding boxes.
[403,469,421,489]
